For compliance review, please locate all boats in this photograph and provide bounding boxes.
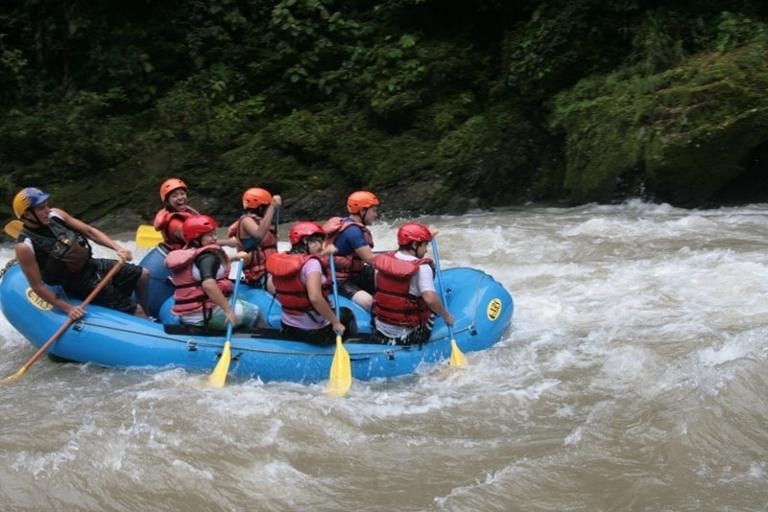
[0,266,513,386]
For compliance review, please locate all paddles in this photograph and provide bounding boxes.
[1,251,128,386]
[136,224,165,250]
[328,251,352,396]
[429,230,470,369]
[206,252,246,387]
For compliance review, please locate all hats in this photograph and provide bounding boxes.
[31,192,50,206]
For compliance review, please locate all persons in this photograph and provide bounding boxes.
[317,191,380,312]
[155,178,200,236]
[12,187,154,320]
[164,216,265,329]
[371,223,455,346]
[230,189,280,298]
[267,221,358,347]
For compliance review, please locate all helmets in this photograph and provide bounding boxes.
[398,224,434,245]
[183,215,217,243]
[160,178,187,201]
[289,221,326,247]
[14,187,44,218]
[243,187,272,210]
[348,191,378,213]
